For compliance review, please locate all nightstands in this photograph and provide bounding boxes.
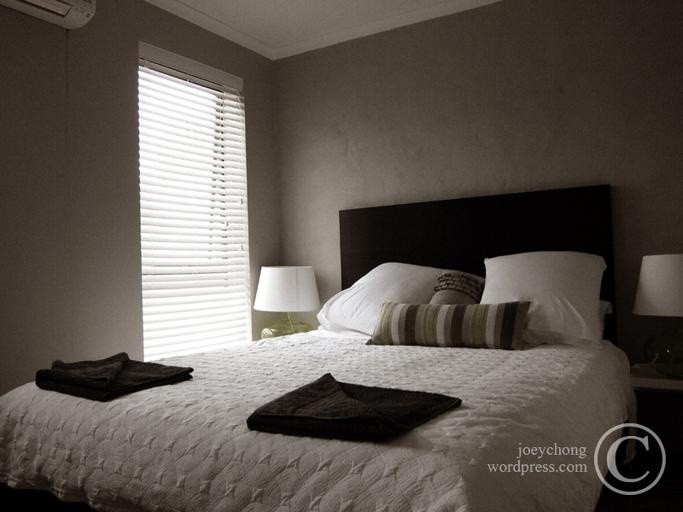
[633,365,682,512]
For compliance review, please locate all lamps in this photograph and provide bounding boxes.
[629,253,682,373]
[253,266,322,335]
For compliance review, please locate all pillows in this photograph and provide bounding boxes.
[597,295,612,339]
[366,302,533,350]
[315,290,349,331]
[316,262,479,339]
[431,271,482,311]
[480,251,609,345]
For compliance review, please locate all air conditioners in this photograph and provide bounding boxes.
[0,0,97,29]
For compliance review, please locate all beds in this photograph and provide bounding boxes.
[2,183,632,512]
